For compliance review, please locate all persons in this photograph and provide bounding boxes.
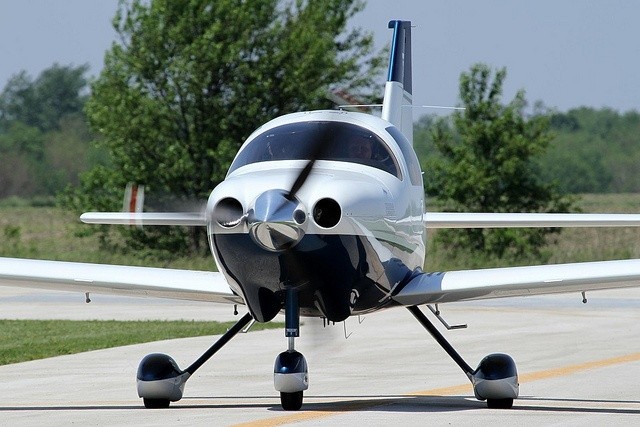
[346,133,374,160]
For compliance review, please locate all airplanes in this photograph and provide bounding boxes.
[79,22,640,408]
